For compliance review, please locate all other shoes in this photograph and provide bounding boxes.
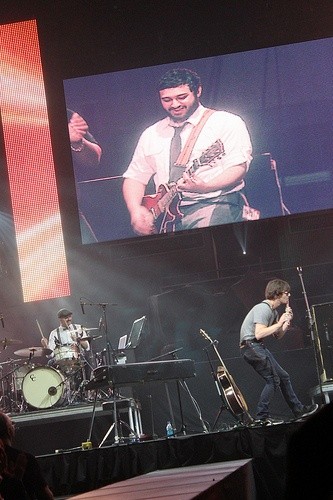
[301,404,318,417]
[254,417,284,425]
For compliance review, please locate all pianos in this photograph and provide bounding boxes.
[85,359,197,446]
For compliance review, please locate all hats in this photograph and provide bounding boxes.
[57,308,72,318]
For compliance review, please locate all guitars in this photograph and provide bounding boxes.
[199,327,248,416]
[141,138,226,234]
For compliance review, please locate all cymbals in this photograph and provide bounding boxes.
[68,328,98,332]
[80,336,102,341]
[14,346,53,357]
[0,339,21,346]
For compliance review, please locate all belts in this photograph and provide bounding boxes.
[241,338,261,345]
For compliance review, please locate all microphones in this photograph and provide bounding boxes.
[0,312,4,328]
[80,300,85,315]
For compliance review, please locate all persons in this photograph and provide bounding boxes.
[66,69,255,246]
[40,309,91,402]
[239,279,319,425]
[0,413,54,500]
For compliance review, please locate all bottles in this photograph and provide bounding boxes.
[166,422,173,439]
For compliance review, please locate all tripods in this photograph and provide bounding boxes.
[0,307,142,448]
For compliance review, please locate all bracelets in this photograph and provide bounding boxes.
[285,322,290,326]
[70,138,85,152]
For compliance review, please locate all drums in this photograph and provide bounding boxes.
[22,365,71,409]
[54,343,81,365]
[11,362,40,393]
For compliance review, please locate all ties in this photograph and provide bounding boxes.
[169,122,188,177]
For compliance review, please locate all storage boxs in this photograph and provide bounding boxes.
[96,316,147,367]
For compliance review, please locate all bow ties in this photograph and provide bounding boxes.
[63,327,71,331]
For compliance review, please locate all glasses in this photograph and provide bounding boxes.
[284,292,288,296]
